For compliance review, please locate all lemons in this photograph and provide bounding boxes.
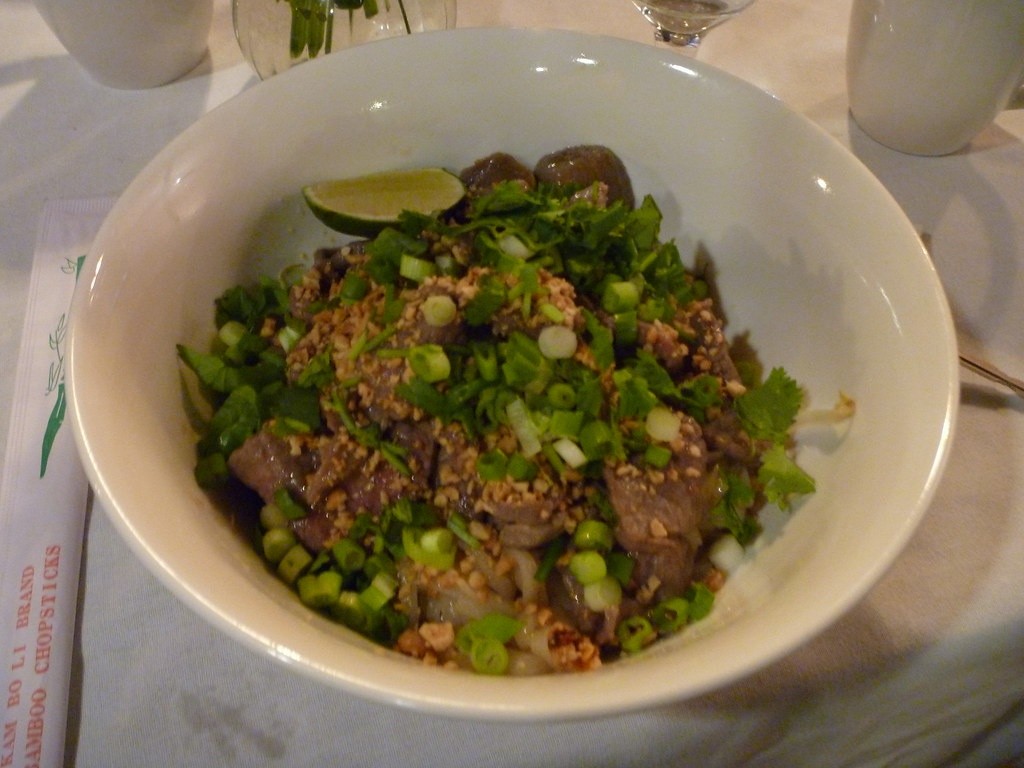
[295,166,467,236]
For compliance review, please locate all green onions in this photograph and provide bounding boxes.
[188,218,748,675]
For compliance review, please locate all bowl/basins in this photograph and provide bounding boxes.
[64,27,958,721]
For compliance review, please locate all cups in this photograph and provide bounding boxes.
[846,0,1024,157]
[32,0,214,89]
[631,0,756,55]
